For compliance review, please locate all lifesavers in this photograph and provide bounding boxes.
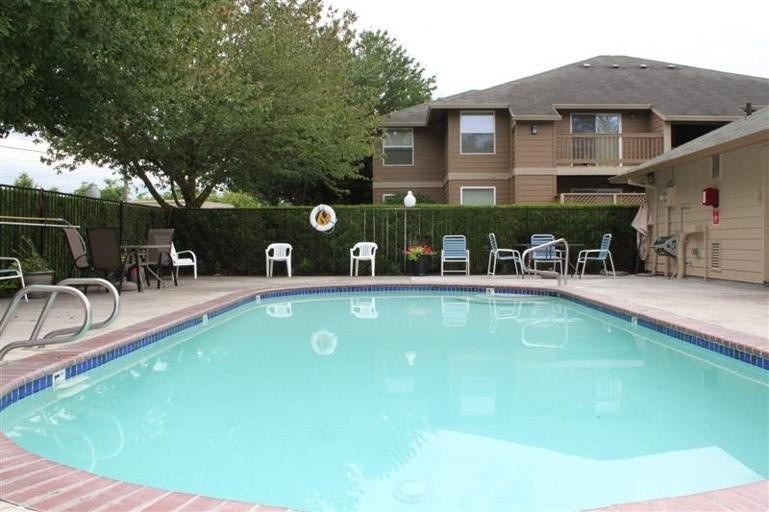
[310,204,336,231]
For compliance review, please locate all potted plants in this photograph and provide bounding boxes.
[13,234,56,298]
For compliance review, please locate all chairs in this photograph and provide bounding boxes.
[0,257,29,306]
[350,242,378,277]
[62,227,90,278]
[487,233,524,279]
[440,234,470,277]
[84,227,141,296]
[574,233,615,278]
[170,240,197,281]
[265,243,292,278]
[142,228,175,288]
[530,234,563,275]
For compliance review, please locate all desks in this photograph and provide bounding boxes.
[121,245,171,291]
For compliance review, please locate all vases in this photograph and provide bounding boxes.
[400,256,432,276]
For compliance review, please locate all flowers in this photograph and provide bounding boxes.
[402,244,436,261]
